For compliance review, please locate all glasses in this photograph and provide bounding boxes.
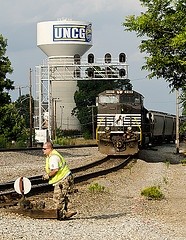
[42,148,47,150]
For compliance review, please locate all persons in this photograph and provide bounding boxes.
[42,142,77,221]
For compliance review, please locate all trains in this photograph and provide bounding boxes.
[97,90,181,156]
